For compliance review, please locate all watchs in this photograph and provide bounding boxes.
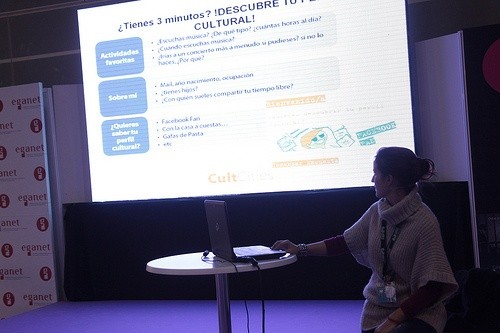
[298,243,308,257]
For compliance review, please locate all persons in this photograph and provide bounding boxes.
[269,146,459,333]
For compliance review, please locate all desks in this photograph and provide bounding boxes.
[146,248,298,333]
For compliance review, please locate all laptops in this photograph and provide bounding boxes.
[204,199,285,263]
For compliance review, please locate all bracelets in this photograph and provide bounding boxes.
[387,316,399,324]
